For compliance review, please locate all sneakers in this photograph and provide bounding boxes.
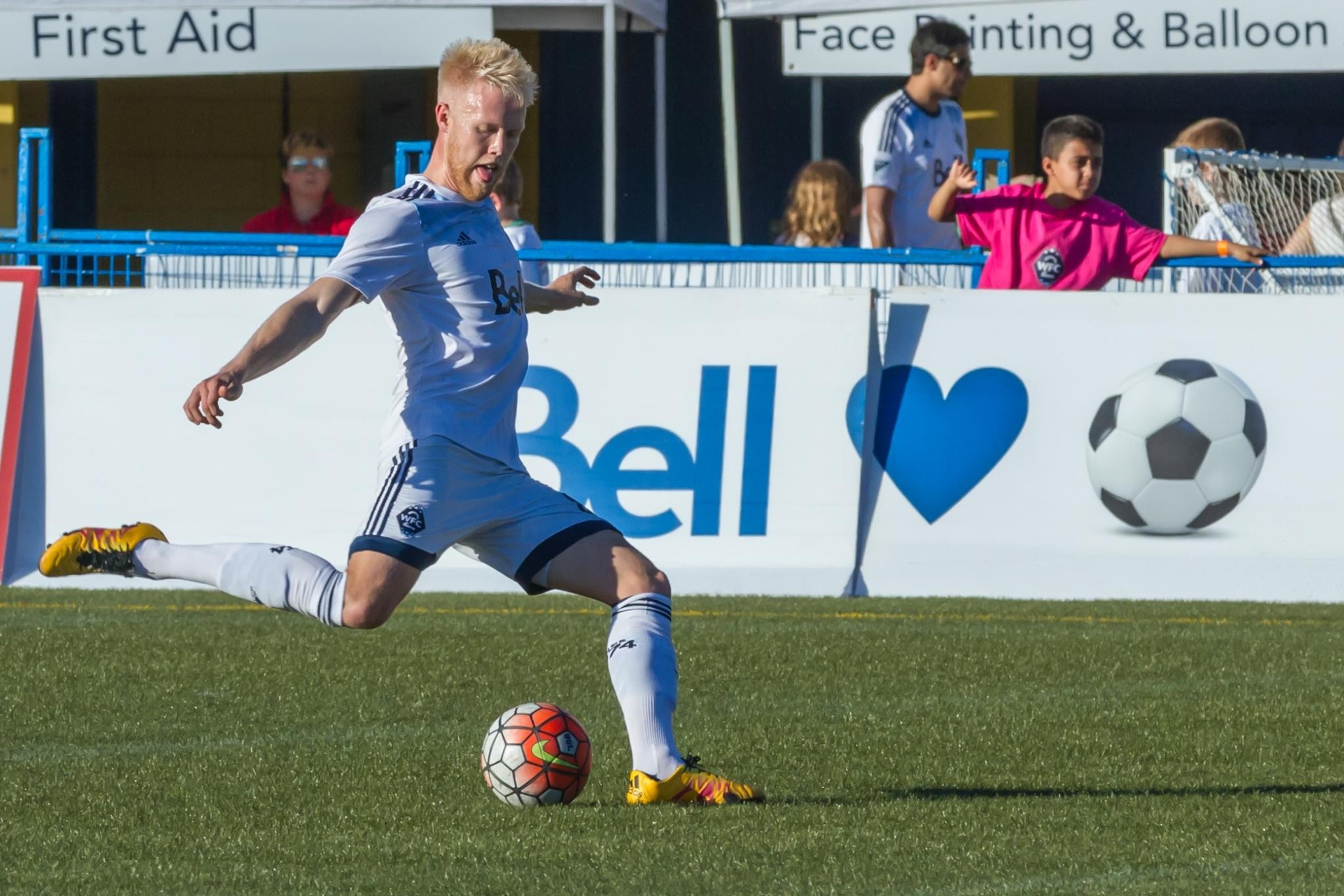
[623,752,768,808]
[35,521,168,581]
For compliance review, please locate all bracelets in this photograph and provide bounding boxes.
[1216,241,1230,256]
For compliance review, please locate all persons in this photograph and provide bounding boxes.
[927,114,1276,290]
[1278,138,1344,254]
[1172,120,1265,295]
[857,21,972,252]
[36,38,767,805]
[777,159,859,246]
[488,155,551,288]
[241,130,363,236]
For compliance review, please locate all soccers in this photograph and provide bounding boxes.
[1084,356,1268,536]
[480,701,593,808]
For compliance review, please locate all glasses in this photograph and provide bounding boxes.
[284,156,335,173]
[922,48,973,71]
